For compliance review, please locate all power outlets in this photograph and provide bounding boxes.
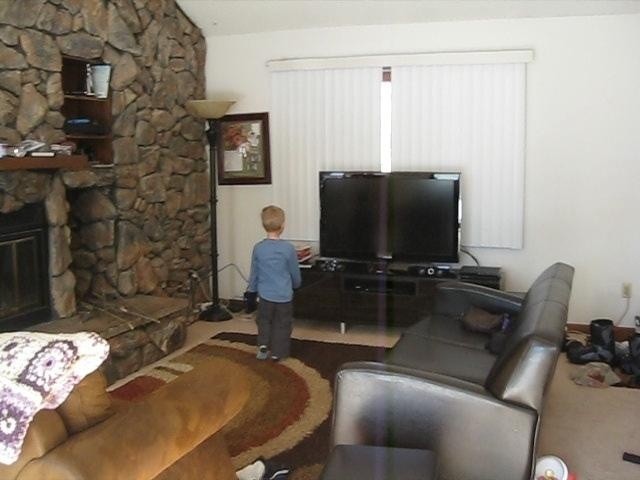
[623,283,631,297]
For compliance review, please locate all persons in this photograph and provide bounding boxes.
[246,206,302,362]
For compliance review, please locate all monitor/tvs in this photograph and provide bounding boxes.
[319,171,460,276]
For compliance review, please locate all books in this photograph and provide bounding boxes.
[0,139,75,158]
[89,64,112,99]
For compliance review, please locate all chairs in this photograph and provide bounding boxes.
[0,330,248,480]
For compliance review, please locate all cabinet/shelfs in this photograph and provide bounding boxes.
[291,261,504,337]
[59,55,114,168]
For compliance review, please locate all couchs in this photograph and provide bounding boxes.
[329,261,576,480]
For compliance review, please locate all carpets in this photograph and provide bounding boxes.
[106,332,393,480]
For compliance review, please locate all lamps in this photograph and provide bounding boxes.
[186,99,238,322]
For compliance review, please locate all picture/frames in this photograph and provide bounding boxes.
[214,113,271,186]
